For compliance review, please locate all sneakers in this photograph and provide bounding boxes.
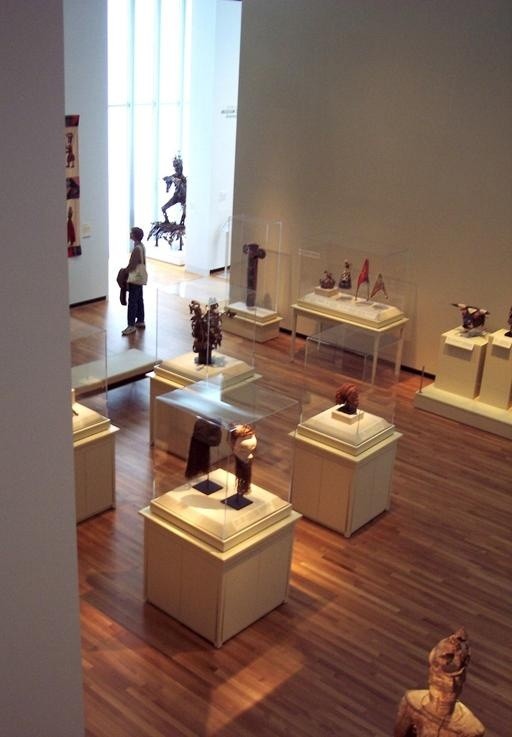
[135,322,145,329]
[121,325,136,335]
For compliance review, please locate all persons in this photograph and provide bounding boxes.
[391,629,486,737]
[162,158,187,225]
[121,227,148,336]
[228,421,258,496]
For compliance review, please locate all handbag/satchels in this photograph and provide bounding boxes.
[127,263,148,285]
